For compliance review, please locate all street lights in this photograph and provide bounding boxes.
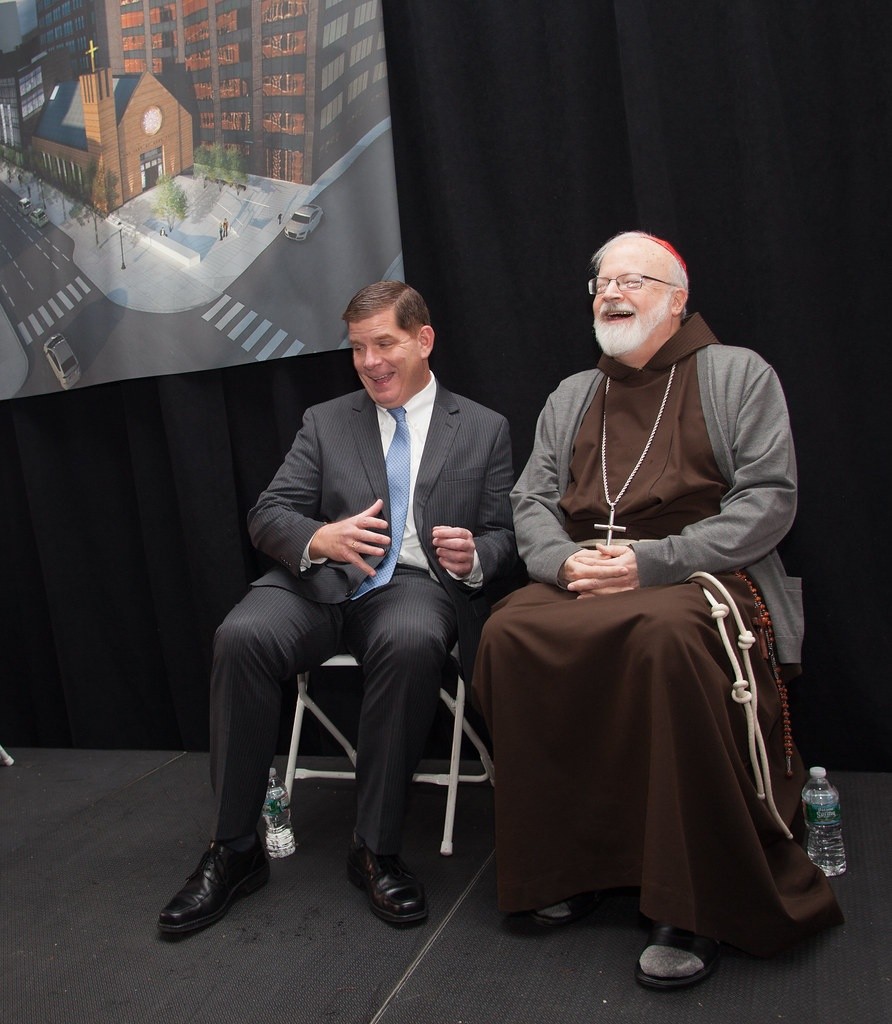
[37,176,47,210]
[117,222,126,270]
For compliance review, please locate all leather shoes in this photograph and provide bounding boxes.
[346,843,428,922]
[159,830,268,933]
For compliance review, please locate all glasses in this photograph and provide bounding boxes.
[588,273,682,294]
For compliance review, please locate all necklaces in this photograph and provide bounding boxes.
[593,357,685,550]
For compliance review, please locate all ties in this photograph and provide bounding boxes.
[350,407,411,600]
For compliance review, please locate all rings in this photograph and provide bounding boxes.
[351,539,359,554]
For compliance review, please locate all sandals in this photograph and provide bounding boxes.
[528,887,608,927]
[635,925,721,989]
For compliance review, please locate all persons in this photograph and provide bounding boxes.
[218,217,231,243]
[277,211,285,226]
[470,226,799,990]
[154,279,517,948]
[158,226,169,238]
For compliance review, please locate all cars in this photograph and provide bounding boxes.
[16,197,33,215]
[29,208,49,227]
[43,333,82,390]
[282,203,324,242]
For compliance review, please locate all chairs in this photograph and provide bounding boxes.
[285,636,497,857]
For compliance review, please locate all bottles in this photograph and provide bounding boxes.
[802,767,846,876]
[262,767,296,857]
